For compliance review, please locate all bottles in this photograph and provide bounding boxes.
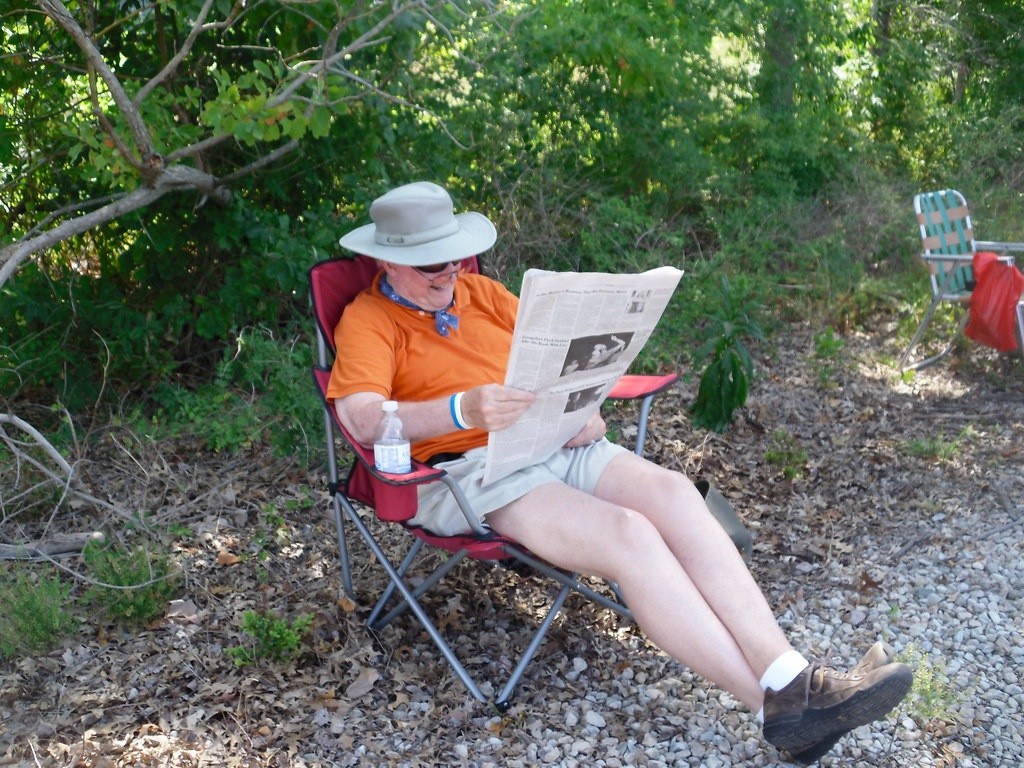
[373,400,412,474]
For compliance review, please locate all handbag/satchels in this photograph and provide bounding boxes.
[964,253,1024,353]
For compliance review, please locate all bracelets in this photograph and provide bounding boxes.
[450,392,474,430]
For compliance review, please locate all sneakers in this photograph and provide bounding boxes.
[761,640,913,766]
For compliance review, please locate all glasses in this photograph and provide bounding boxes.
[410,260,465,289]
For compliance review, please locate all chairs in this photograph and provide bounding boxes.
[897,189,1024,373]
[306,253,680,713]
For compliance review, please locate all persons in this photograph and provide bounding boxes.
[325,180,912,762]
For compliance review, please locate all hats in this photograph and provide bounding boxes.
[339,182,497,266]
